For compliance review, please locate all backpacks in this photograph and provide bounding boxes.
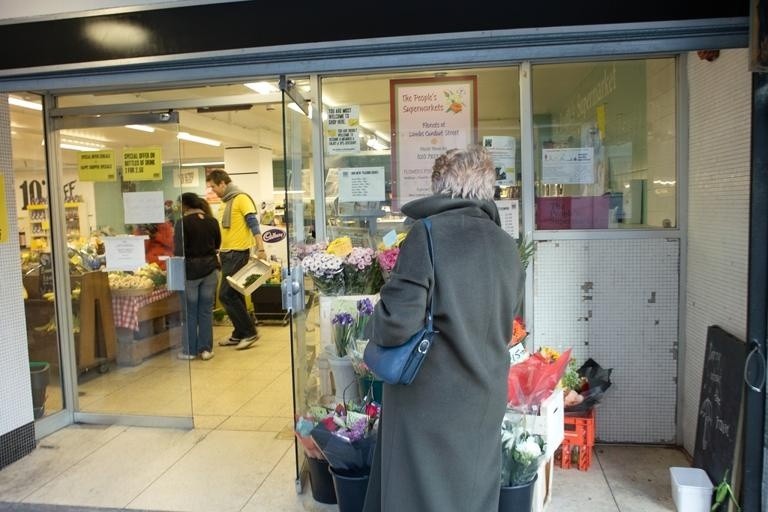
[363,218,440,387]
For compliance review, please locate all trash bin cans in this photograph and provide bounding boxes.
[29,362,49,419]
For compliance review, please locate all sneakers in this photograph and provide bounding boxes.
[177,330,259,360]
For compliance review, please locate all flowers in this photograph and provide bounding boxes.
[291,233,613,486]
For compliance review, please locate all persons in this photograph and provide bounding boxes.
[171,191,222,362]
[359,142,528,512]
[205,169,270,351]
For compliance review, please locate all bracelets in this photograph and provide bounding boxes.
[256,249,265,252]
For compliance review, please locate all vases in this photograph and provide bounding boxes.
[499,474,538,512]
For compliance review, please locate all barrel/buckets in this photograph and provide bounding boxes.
[498,469,539,512]
[357,377,384,403]
[328,357,357,399]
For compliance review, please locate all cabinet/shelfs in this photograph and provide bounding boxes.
[111,285,184,367]
[22,269,117,376]
[27,202,89,252]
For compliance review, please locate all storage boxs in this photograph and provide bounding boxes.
[226,254,272,296]
[157,255,186,290]
[537,197,609,229]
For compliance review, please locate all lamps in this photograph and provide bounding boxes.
[244,82,390,151]
[9,95,225,148]
[38,138,112,152]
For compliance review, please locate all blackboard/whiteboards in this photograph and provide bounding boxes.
[692,325,747,512]
